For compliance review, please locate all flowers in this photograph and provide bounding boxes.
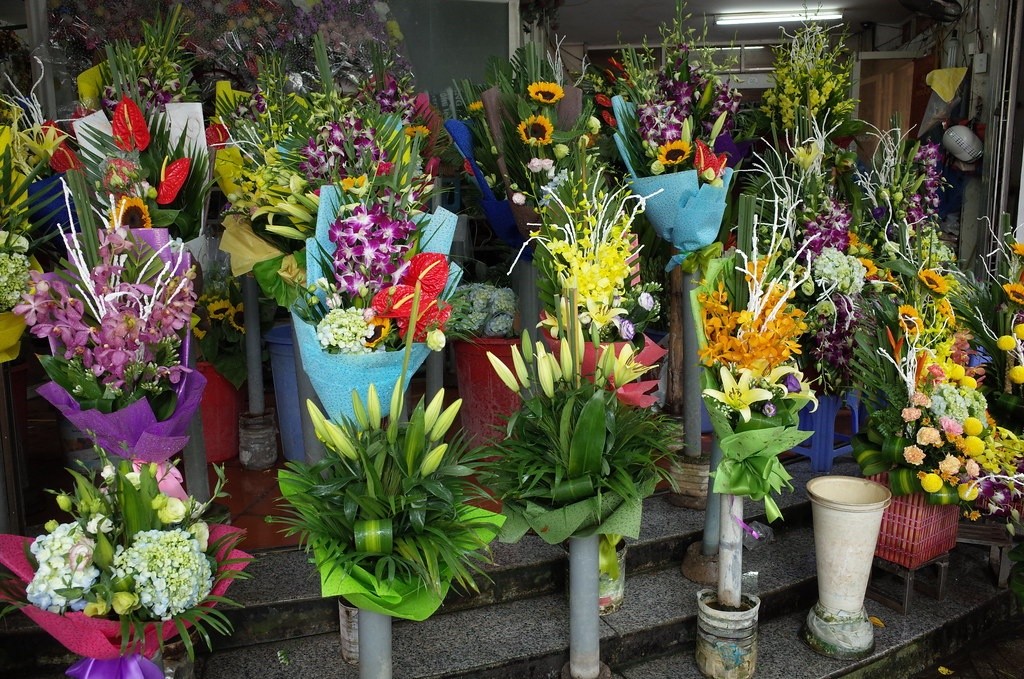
[1,0,1024,668]
